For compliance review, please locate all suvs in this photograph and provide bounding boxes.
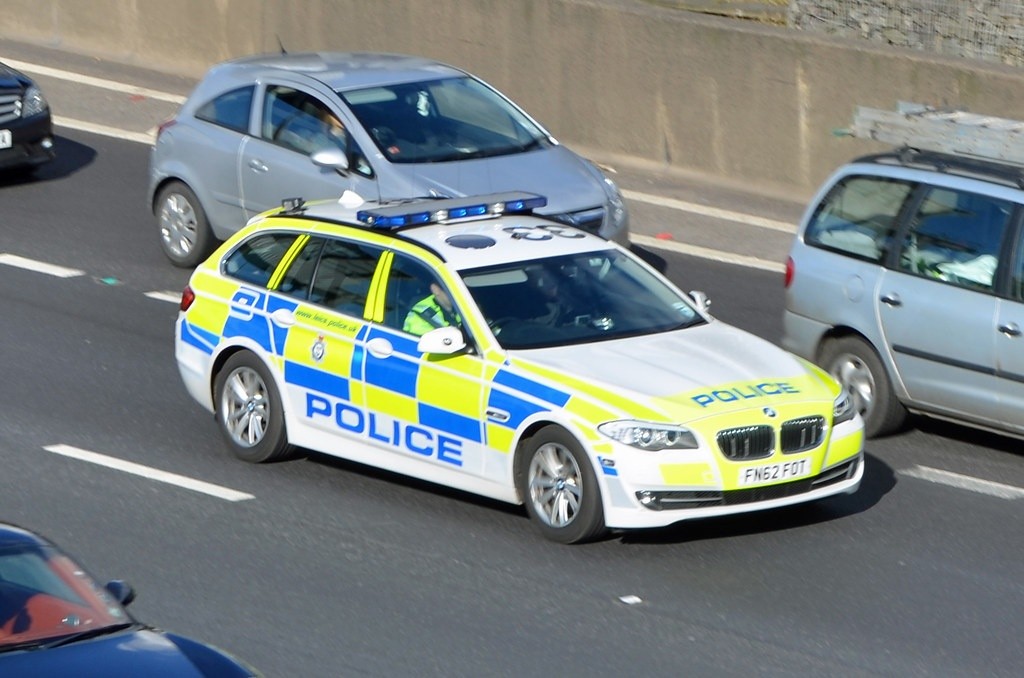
[777,141,1023,441]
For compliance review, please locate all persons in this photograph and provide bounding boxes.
[403,279,500,354]
[312,109,348,152]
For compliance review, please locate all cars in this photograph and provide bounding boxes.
[148,49,630,267]
[174,189,866,545]
[0,59,56,169]
[0,521,267,678]
